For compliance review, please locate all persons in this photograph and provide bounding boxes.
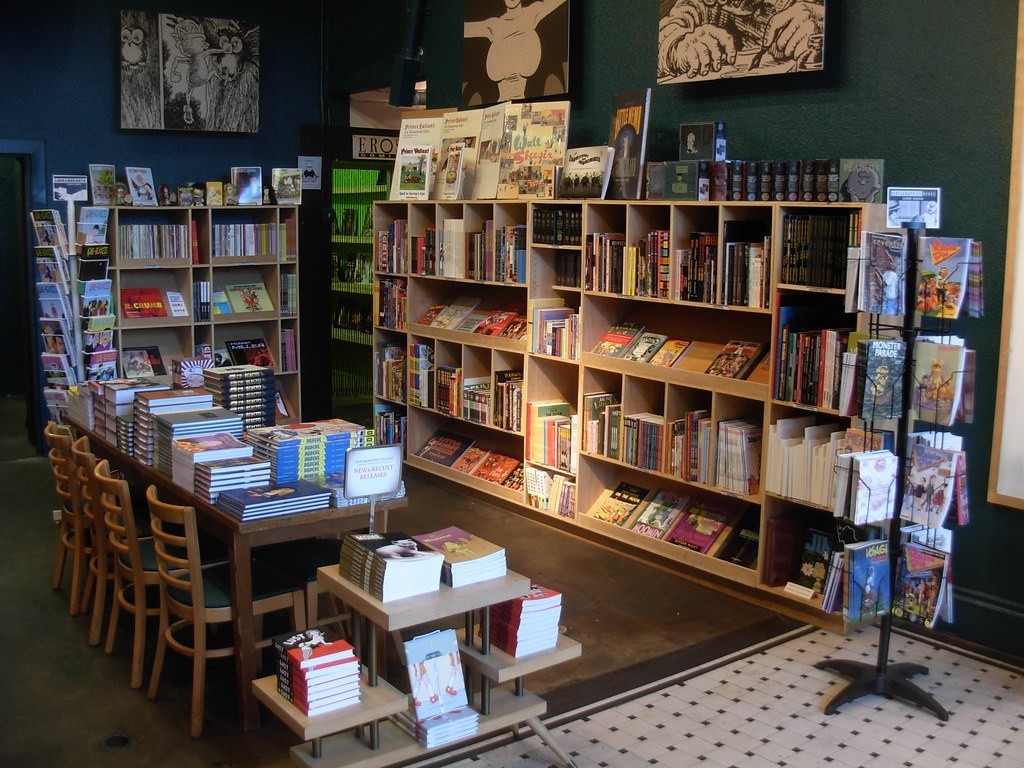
[262,186,270,203]
[158,184,173,206]
[191,182,205,207]
[224,183,237,206]
[115,182,128,204]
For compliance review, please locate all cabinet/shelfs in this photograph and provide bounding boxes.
[251,560,583,768]
[32,201,117,408]
[331,160,393,429]
[814,187,986,722]
[372,199,912,636]
[108,197,302,425]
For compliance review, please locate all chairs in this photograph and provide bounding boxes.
[71,436,187,646]
[94,459,230,690]
[44,422,152,615]
[146,485,306,737]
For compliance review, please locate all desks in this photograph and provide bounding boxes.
[64,405,407,730]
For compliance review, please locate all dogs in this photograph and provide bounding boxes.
[375,539,434,560]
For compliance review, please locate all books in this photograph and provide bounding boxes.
[774,304,855,410]
[66,357,406,522]
[585,219,771,309]
[88,164,303,205]
[763,518,840,595]
[30,205,117,426]
[118,220,298,419]
[704,339,761,379]
[374,218,526,493]
[527,298,581,361]
[387,87,885,203]
[820,230,985,630]
[275,525,562,749]
[525,399,578,474]
[532,208,582,287]
[590,319,694,368]
[525,467,577,519]
[780,212,862,290]
[764,415,846,509]
[581,392,762,496]
[594,482,760,568]
